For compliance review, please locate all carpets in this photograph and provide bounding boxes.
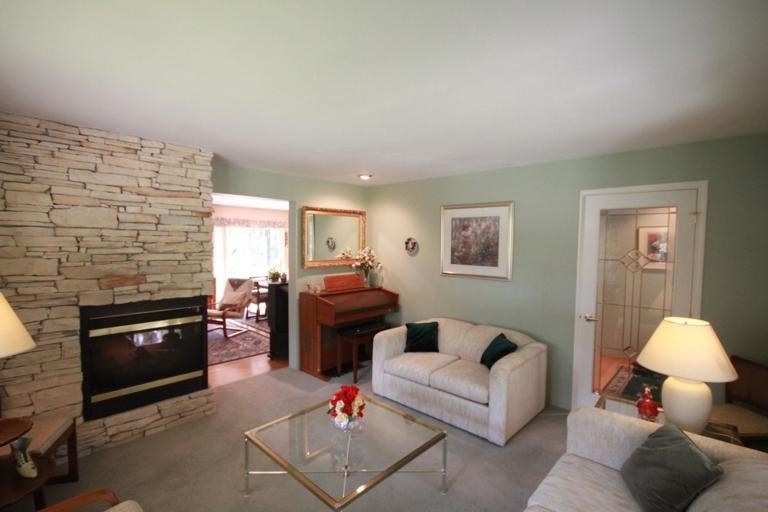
[601,365,668,408]
[208,312,270,368]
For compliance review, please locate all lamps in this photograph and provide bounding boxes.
[636,317,738,434]
[0,293,33,443]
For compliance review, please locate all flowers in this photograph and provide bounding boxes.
[326,385,368,421]
[342,247,379,279]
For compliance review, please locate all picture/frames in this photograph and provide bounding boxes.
[637,226,667,271]
[439,201,515,281]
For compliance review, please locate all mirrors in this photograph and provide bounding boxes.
[302,205,367,269]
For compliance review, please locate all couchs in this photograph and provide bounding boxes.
[524,407,768,512]
[371,317,547,446]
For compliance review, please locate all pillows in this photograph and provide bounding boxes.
[405,322,439,352]
[480,333,518,370]
[620,421,724,511]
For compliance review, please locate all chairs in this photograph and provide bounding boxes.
[207,278,254,339]
[709,355,768,439]
[246,277,269,321]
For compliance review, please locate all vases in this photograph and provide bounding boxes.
[330,417,366,432]
[359,269,370,288]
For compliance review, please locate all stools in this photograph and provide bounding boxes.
[336,322,390,384]
[1,415,78,510]
